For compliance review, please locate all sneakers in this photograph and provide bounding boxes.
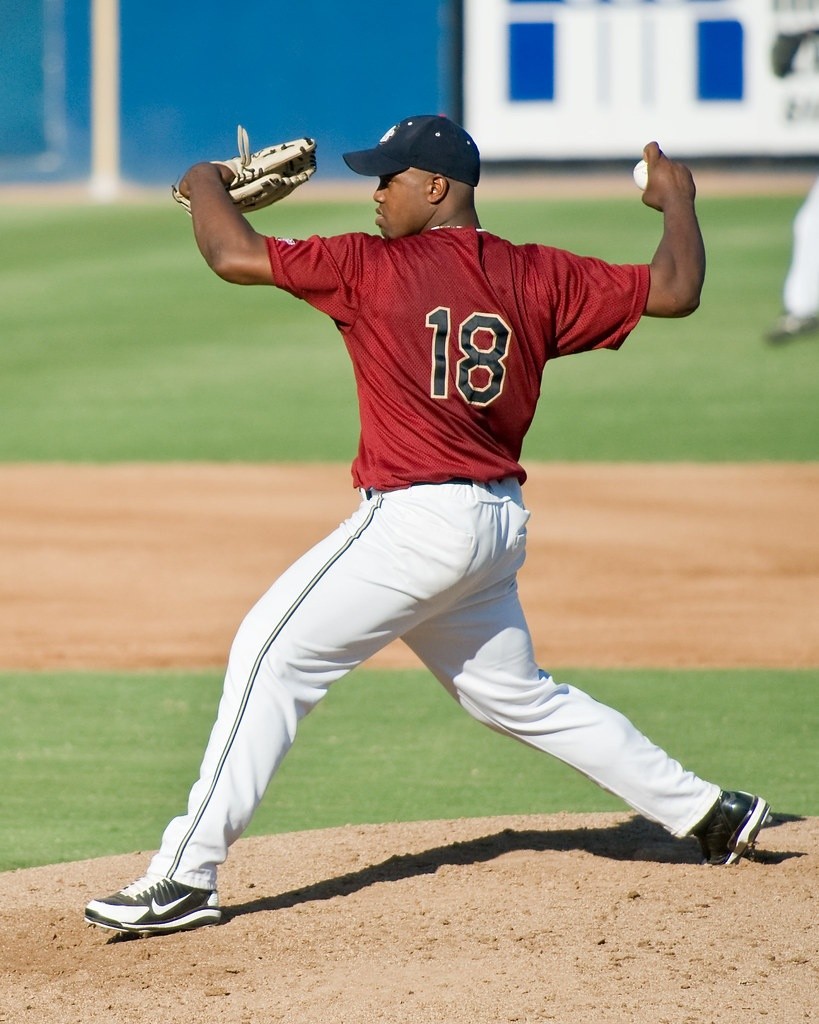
[84,876,222,933]
[692,789,773,865]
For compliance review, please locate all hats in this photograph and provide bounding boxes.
[343,113,480,187]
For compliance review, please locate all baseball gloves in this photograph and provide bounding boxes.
[171,138,319,215]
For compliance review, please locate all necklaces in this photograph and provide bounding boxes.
[431,225,486,232]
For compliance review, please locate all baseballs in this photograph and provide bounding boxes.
[633,157,649,189]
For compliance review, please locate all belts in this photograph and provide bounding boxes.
[366,476,473,500]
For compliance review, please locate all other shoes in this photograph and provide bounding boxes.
[770,314,818,342]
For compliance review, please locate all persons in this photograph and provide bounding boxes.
[767,181,819,341]
[82,115,772,933]
